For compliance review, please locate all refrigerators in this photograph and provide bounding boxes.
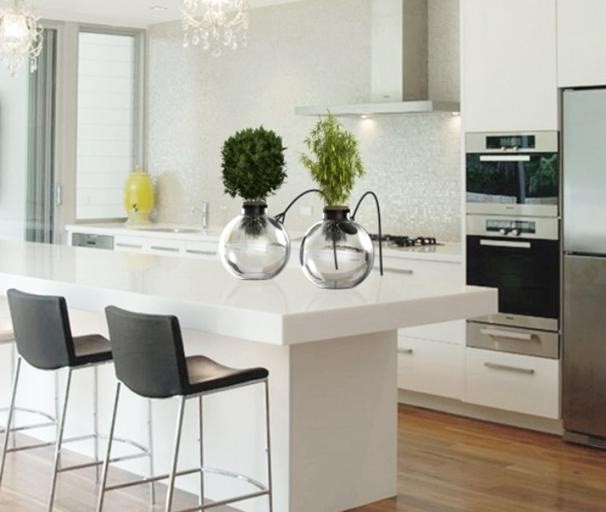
[559,87,606,446]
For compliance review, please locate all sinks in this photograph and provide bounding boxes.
[135,227,200,234]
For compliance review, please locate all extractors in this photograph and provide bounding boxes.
[293,0,460,116]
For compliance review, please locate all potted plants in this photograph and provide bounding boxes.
[218,108,384,292]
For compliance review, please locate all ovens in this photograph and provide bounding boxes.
[463,130,558,362]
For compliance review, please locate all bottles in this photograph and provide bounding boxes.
[219,204,376,290]
[126,165,154,225]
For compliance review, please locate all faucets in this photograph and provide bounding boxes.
[192,200,208,228]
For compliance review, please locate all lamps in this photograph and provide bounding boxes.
[1,0,45,76]
[180,0,253,57]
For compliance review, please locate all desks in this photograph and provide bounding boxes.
[2,243,504,511]
[2,289,273,512]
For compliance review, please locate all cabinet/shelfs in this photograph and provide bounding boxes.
[73,236,563,437]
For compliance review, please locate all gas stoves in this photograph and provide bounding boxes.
[368,233,444,254]
